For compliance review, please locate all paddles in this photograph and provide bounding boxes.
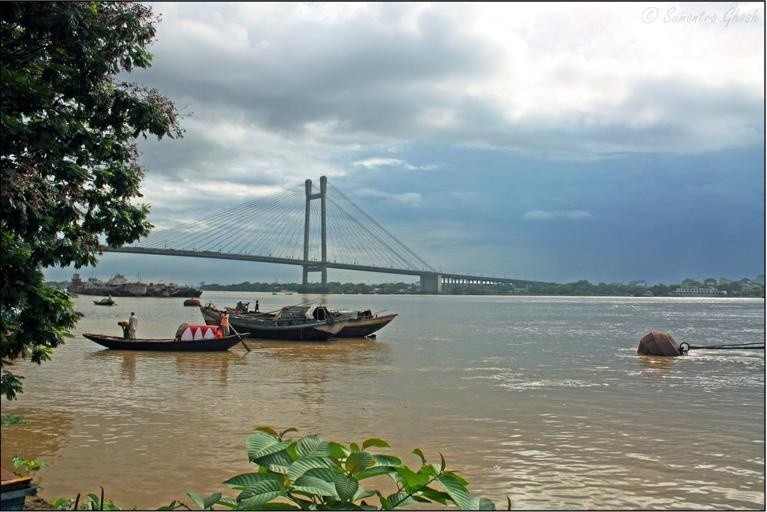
[217,312,252,352]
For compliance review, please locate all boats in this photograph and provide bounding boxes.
[81,323,252,352]
[92,294,115,306]
[331,307,399,339]
[184,299,200,306]
[67,269,203,297]
[199,301,350,342]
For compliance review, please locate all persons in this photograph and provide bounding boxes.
[221,310,230,336]
[128,311,138,341]
[255,299,259,312]
[118,320,129,338]
[236,301,250,313]
